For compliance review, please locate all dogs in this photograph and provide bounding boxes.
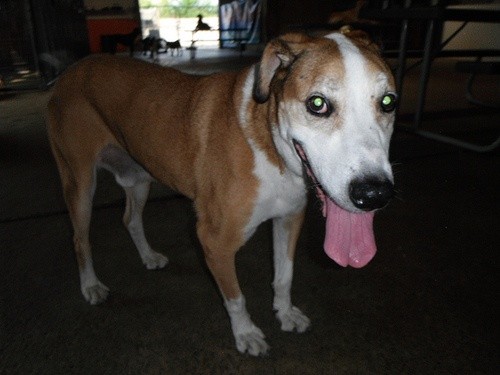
[39,14,399,357]
